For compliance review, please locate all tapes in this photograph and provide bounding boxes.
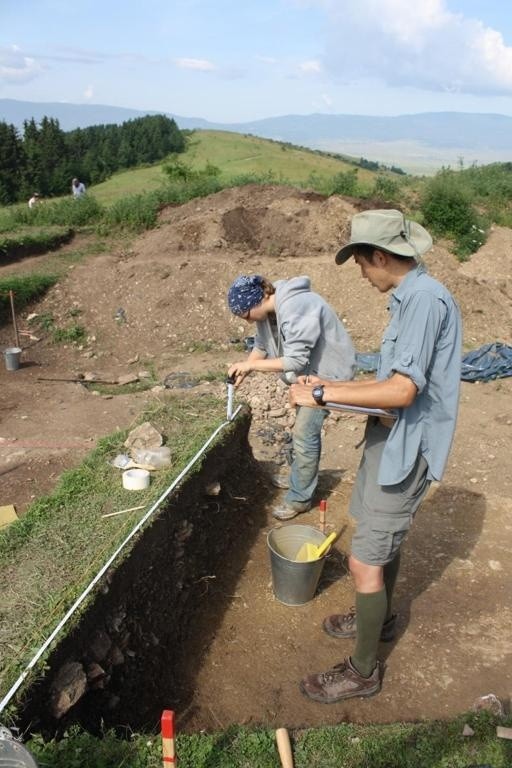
[121,468,151,491]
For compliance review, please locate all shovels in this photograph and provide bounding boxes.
[292,530,339,564]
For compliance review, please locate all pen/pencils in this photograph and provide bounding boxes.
[306,363,310,386]
[101,504,147,519]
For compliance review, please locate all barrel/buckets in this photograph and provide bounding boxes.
[4,347,22,371]
[267,524,332,607]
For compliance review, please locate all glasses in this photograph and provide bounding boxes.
[242,308,251,320]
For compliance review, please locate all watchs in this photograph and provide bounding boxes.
[312,383,327,407]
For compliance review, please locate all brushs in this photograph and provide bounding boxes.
[112,453,157,472]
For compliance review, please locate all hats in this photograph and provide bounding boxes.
[334,209,434,266]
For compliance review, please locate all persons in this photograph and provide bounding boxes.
[288,204,464,703]
[72,178,86,196]
[28,192,42,208]
[222,273,358,519]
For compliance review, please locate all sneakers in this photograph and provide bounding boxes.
[299,656,382,703]
[272,500,301,521]
[270,473,290,489]
[323,612,396,642]
